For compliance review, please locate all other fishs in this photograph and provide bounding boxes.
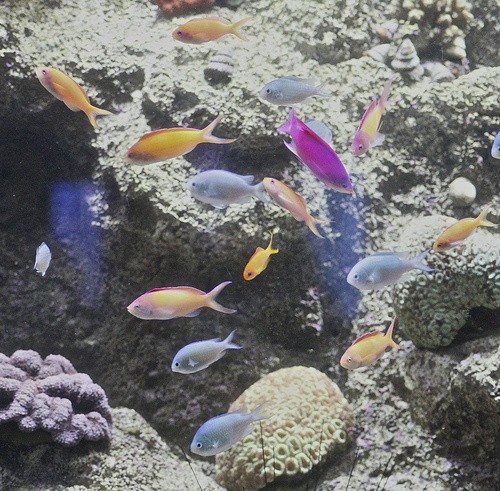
[188,170,268,209]
[351,75,396,156]
[433,205,499,251]
[257,76,334,105]
[35,66,114,131]
[171,328,243,374]
[126,281,237,319]
[190,400,275,456]
[276,107,354,194]
[262,176,335,239]
[346,247,433,290]
[172,15,251,44]
[340,314,402,370]
[123,115,237,166]
[491,132,500,159]
[243,232,278,281]
[34,241,52,277]
